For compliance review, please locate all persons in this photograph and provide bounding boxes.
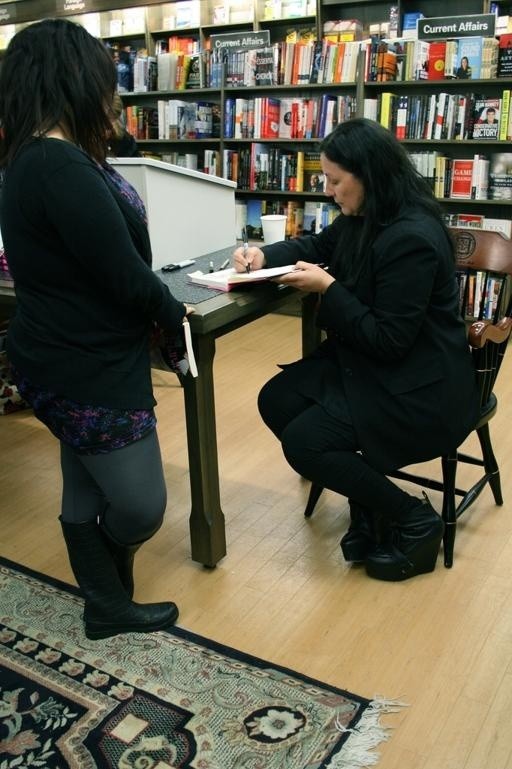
[0,18,199,644]
[103,92,144,157]
[231,115,483,586]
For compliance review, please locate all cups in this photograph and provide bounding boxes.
[260,214,287,246]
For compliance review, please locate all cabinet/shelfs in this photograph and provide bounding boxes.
[0,0,511,327]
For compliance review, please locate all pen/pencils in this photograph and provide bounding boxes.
[161,259,195,271]
[243,229,250,273]
[209,259,230,273]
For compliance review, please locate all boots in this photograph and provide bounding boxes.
[99,511,164,601]
[58,514,179,640]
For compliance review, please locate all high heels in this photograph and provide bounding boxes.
[364,489,445,582]
[340,475,409,563]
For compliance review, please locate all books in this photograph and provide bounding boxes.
[186,261,329,293]
[456,268,504,322]
[148,321,199,381]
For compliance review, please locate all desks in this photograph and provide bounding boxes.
[125,241,326,569]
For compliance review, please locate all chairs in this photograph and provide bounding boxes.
[303,227,512,568]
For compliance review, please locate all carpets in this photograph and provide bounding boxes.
[1,552,408,769]
